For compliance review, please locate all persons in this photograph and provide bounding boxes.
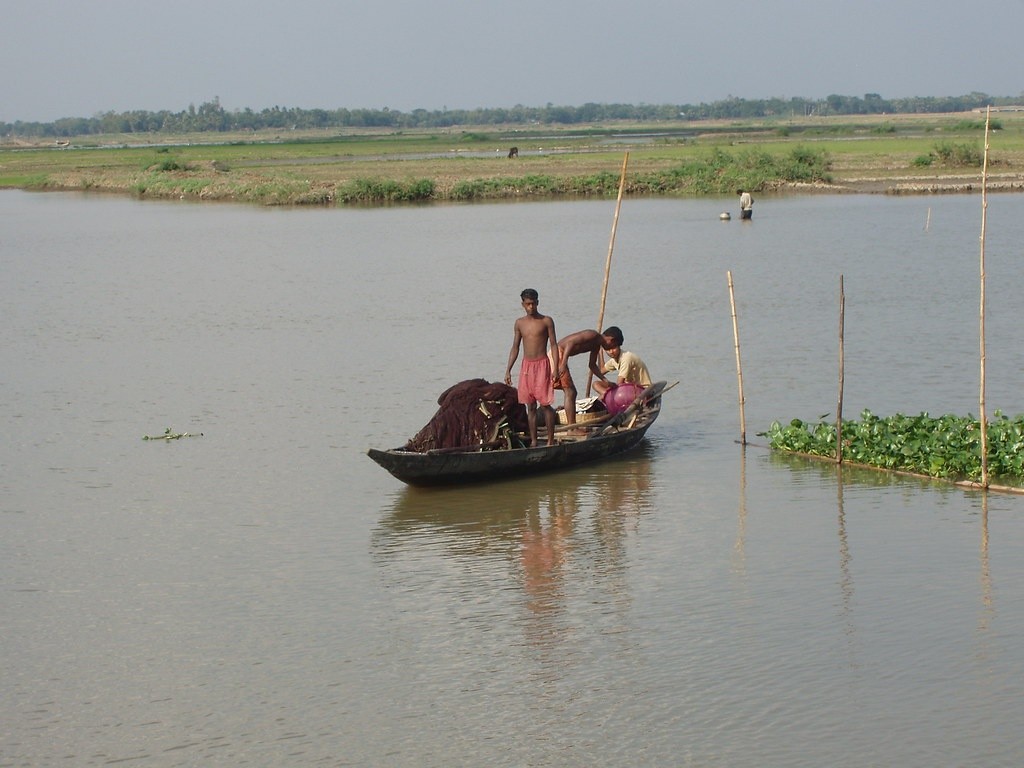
[547,326,625,435]
[593,346,652,406]
[504,289,560,447]
[736,189,755,219]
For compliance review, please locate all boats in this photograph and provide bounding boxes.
[367,380,682,489]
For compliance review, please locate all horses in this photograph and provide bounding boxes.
[507,147,518,160]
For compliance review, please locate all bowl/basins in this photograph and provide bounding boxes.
[601,384,645,416]
